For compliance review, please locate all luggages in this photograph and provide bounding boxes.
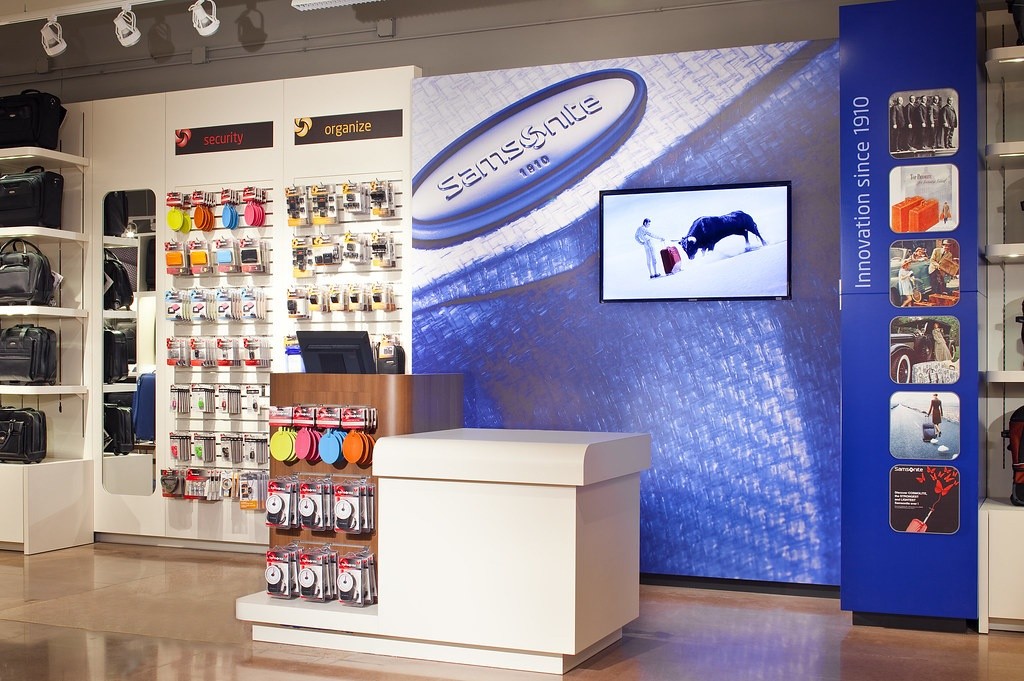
[923,415,935,442]
[104,190,128,236]
[660,241,683,275]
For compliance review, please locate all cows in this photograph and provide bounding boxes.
[677,209,768,266]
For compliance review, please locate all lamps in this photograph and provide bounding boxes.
[40,14,67,57]
[114,3,141,46]
[187,0,221,36]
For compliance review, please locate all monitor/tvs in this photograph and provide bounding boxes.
[297,330,377,373]
[599,180,793,303]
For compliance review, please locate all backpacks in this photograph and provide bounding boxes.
[1002,406,1024,506]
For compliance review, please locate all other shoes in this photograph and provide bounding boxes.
[894,143,956,154]
[935,433,937,438]
[938,431,942,437]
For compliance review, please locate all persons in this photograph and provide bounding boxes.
[932,323,953,361]
[929,240,953,302]
[635,218,665,279]
[942,202,949,223]
[899,247,928,308]
[891,94,958,153]
[927,394,944,439]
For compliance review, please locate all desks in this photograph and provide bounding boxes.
[370,427,652,675]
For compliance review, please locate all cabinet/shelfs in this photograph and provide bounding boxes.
[104,235,142,454]
[0,145,93,553]
[978,46,1024,632]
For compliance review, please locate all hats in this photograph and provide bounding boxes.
[941,239,953,245]
[901,258,911,267]
[944,201,947,204]
[933,394,937,396]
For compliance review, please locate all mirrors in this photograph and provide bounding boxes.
[100,188,159,495]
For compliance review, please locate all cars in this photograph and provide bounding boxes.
[891,318,957,384]
[889,247,953,307]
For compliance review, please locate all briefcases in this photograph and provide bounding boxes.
[939,258,960,277]
[0,324,59,386]
[891,196,940,232]
[913,288,960,308]
[0,405,48,464]
[0,238,58,308]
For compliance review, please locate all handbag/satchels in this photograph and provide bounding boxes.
[0,164,64,229]
[940,213,944,219]
[103,238,156,456]
[1,89,67,149]
